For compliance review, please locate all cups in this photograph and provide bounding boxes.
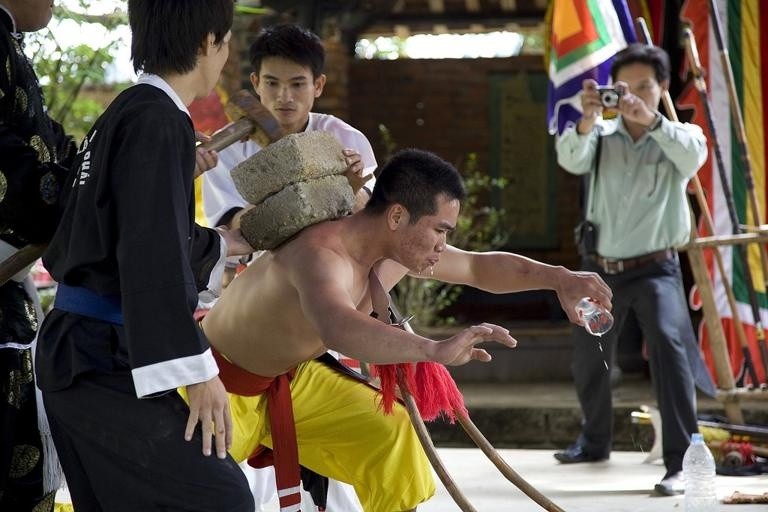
[576,296,614,337]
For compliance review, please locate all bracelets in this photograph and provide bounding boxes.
[646,106,664,136]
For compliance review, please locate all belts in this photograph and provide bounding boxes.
[589,248,673,276]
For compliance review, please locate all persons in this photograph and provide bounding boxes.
[200,20,385,512]
[553,41,710,496]
[1,1,220,512]
[55,147,615,512]
[34,1,260,512]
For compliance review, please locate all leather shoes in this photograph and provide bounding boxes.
[655,466,685,496]
[554,445,609,463]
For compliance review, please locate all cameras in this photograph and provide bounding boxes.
[591,85,620,108]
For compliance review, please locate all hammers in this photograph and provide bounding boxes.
[0,88,282,286]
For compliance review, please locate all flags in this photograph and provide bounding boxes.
[538,0,646,142]
[676,3,768,390]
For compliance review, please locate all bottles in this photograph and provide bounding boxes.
[679,433,718,512]
[724,434,752,466]
